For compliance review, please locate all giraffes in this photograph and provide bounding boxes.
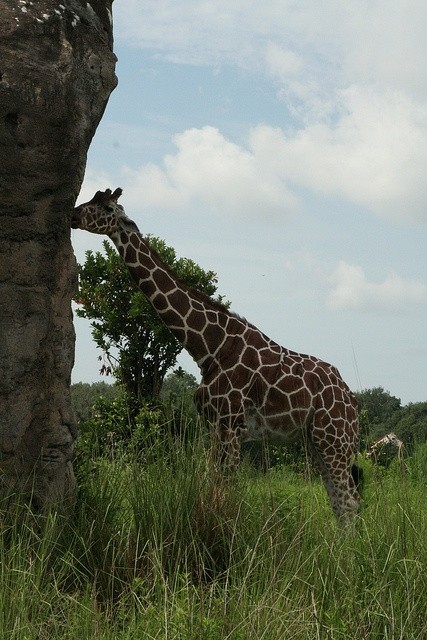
[72,188,363,592]
